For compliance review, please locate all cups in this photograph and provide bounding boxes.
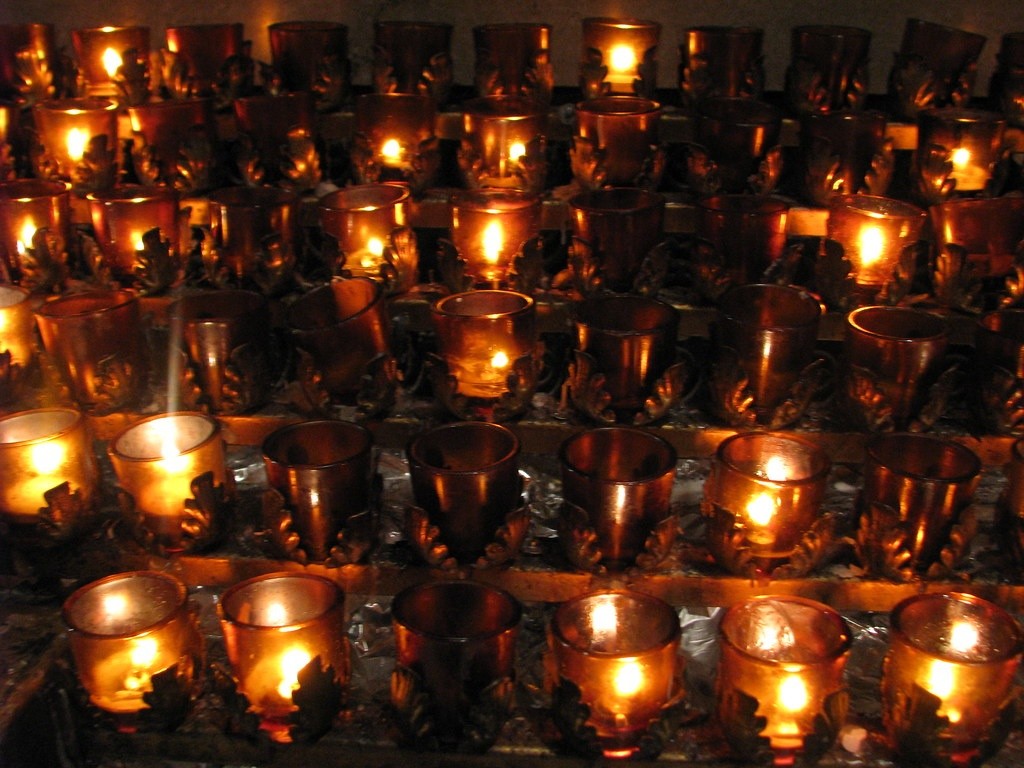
[1,20,1024,768]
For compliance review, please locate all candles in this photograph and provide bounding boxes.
[0,16,1024,768]
